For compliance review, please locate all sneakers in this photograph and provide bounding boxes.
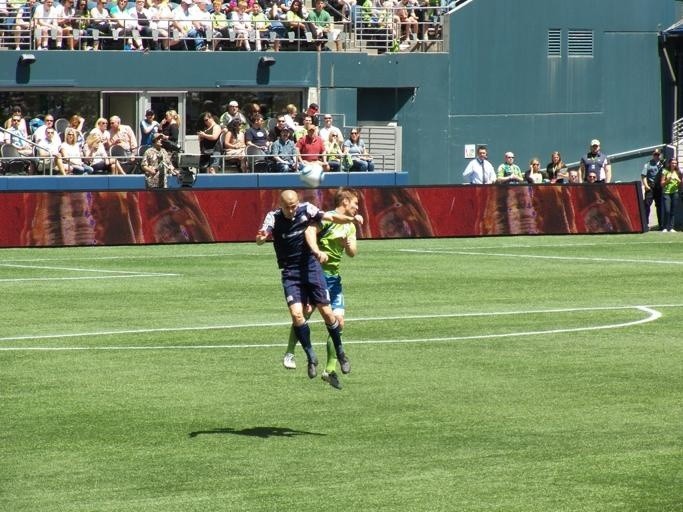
[307,353,318,378]
[321,369,343,390]
[283,351,296,369]
[336,350,350,373]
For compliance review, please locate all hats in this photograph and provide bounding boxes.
[146,109,154,115]
[651,149,661,155]
[152,133,164,143]
[310,103,319,114]
[282,127,290,133]
[590,139,600,146]
[181,0,193,5]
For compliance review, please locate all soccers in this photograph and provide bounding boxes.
[300,163,325,188]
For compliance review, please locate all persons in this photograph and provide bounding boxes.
[660,158,682,232]
[642,149,664,233]
[256,190,364,369]
[282,186,360,390]
[462,139,612,185]
[0,102,376,188]
[1,0,463,53]
